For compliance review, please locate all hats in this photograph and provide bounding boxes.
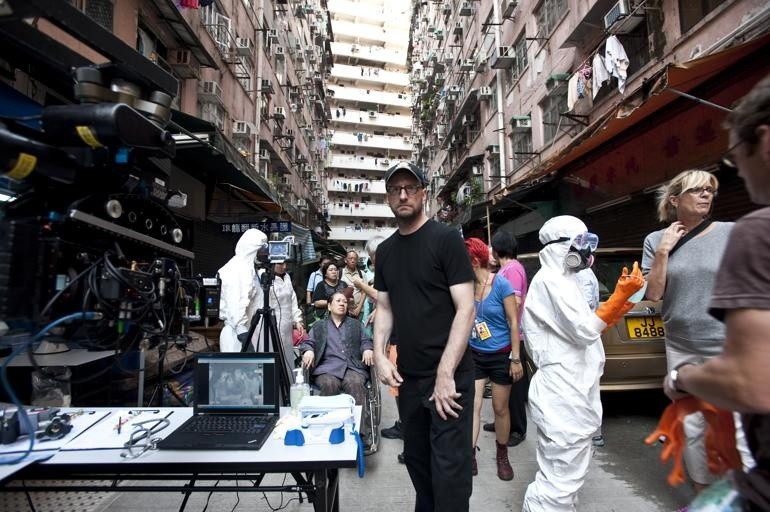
[384,160,428,189]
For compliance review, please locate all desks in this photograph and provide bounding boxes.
[0,405,363,512]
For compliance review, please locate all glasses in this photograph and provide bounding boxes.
[683,185,715,195]
[719,133,757,169]
[578,231,600,252]
[386,185,424,195]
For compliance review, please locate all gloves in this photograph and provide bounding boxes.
[235,331,256,351]
[700,400,746,482]
[596,261,649,331]
[643,383,705,488]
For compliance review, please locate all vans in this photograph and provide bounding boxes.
[510,247,672,392]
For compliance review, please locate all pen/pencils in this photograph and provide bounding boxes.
[113,417,128,434]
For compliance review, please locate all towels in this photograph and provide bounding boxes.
[298,392,356,426]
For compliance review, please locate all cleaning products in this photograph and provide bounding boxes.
[288,366,311,414]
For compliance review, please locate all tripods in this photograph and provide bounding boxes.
[238,273,302,407]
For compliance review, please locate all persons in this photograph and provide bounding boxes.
[220,226,606,481]
[521,213,649,511]
[663,80,770,510]
[640,168,756,494]
[372,163,479,512]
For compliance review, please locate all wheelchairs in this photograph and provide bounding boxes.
[299,357,384,456]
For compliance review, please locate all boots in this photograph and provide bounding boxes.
[471,446,480,477]
[494,439,515,482]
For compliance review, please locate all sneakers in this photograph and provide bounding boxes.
[395,450,405,463]
[483,420,496,432]
[509,429,527,445]
[483,385,493,398]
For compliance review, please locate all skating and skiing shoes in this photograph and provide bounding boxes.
[379,418,402,439]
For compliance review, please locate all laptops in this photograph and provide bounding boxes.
[157,351,280,451]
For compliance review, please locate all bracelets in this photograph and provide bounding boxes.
[667,360,697,395]
